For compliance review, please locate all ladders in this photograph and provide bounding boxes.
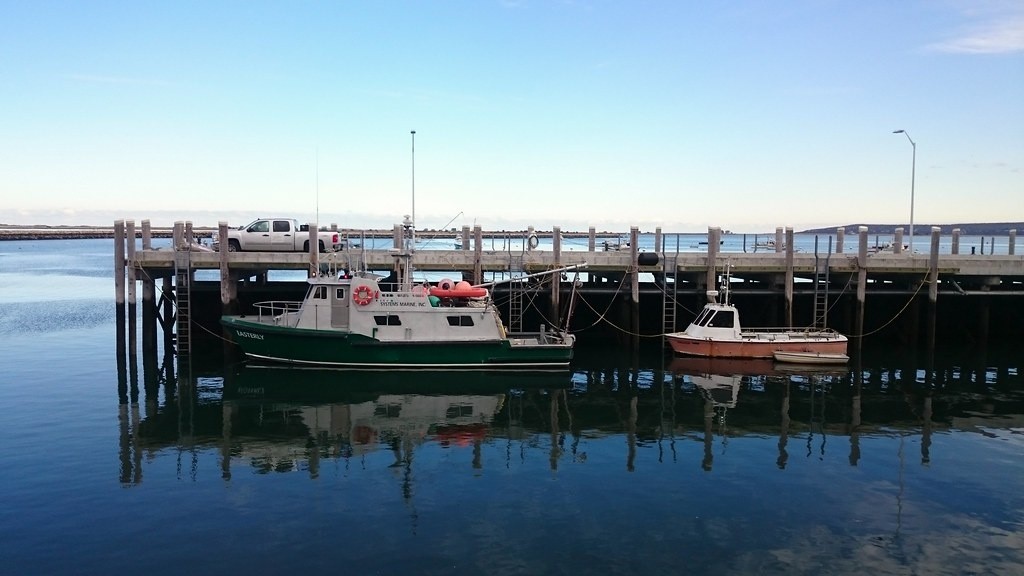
[173,225,192,358]
[346,231,365,278]
[812,235,832,327]
[508,234,524,332]
[662,233,680,333]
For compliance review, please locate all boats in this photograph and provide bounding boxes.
[752,236,791,250]
[666,255,849,363]
[602,234,644,251]
[670,354,850,457]
[882,241,909,251]
[453,232,464,248]
[414,235,422,242]
[699,239,724,245]
[219,148,576,375]
[224,369,572,528]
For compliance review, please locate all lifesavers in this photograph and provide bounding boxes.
[353,284,372,306]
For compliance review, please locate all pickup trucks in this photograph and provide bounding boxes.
[213,216,341,252]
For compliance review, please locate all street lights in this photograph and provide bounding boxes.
[892,129,916,249]
